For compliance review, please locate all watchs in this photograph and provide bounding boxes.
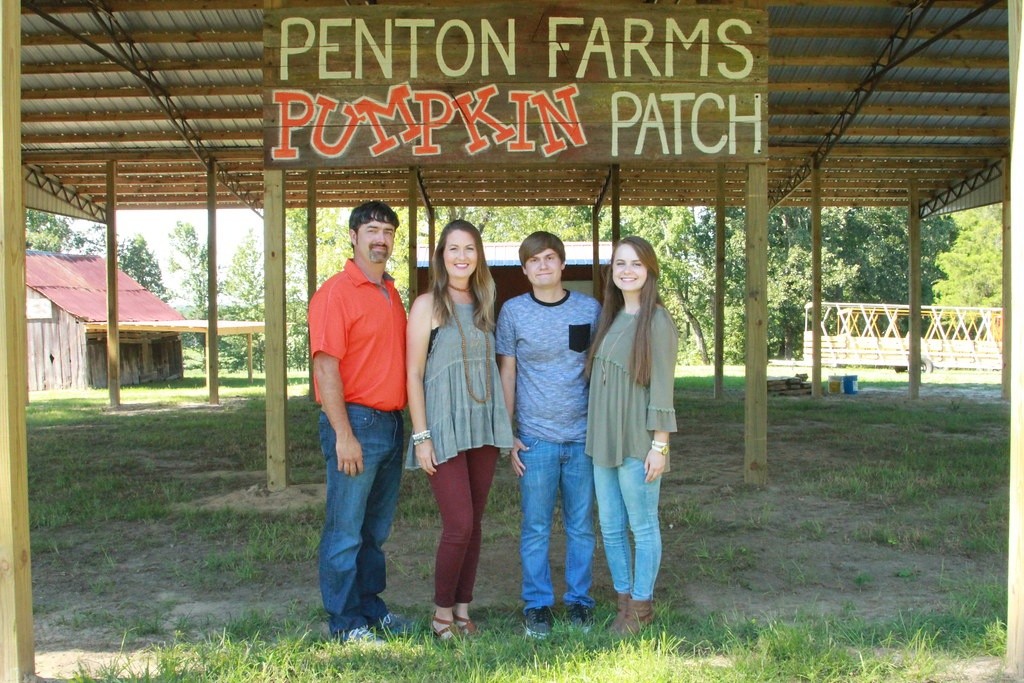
[652,440,669,455]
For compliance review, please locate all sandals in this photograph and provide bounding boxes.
[429,609,483,651]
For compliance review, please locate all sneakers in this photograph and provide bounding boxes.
[564,601,596,636]
[333,611,419,656]
[521,607,552,640]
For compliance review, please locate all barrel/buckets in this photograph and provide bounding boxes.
[842,375,857,394]
[828,375,842,393]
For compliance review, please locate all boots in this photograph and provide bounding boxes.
[605,593,655,638]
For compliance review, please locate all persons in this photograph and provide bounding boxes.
[496,231,604,638]
[585,236,678,633]
[308,201,408,642]
[404,220,513,643]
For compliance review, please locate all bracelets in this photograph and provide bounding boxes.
[412,430,431,445]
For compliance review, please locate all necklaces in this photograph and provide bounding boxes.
[452,302,490,401]
[601,309,640,385]
[448,284,466,292]
